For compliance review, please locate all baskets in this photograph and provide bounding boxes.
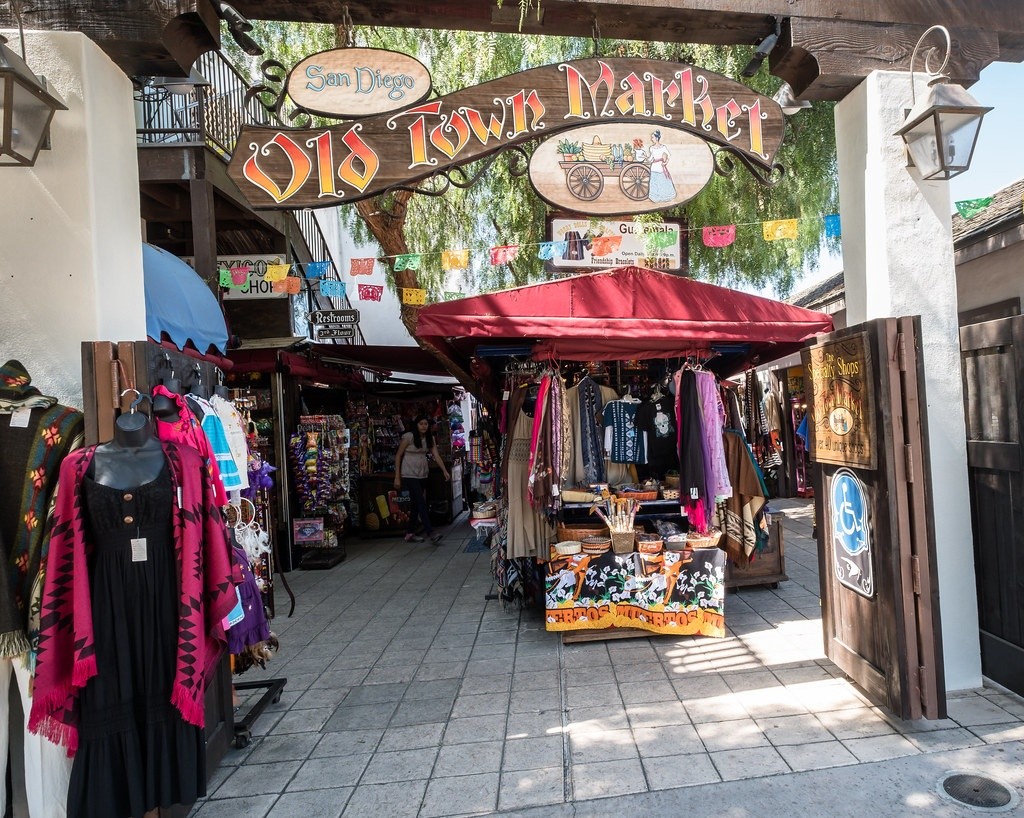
[660,488,680,499]
[555,524,722,553]
[664,469,680,488]
[473,510,495,519]
[616,491,659,500]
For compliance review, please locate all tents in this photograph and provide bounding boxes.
[415,265,834,412]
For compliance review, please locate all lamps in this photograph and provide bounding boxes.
[0,0,70,167]
[891,24,994,182]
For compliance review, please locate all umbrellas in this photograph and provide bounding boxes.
[142,239,229,361]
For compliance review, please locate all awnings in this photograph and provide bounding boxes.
[311,339,456,383]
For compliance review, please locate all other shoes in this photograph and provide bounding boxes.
[405,534,425,543]
[431,534,443,543]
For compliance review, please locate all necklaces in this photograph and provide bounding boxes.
[224,526,269,654]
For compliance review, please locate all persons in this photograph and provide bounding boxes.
[761,387,783,467]
[184,384,242,493]
[394,415,450,544]
[209,386,252,491]
[150,377,228,508]
[0,358,82,815]
[57,410,218,778]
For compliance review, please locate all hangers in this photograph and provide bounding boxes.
[152,351,193,421]
[234,387,258,434]
[91,387,169,454]
[519,356,703,404]
[213,366,230,402]
[185,362,210,421]
